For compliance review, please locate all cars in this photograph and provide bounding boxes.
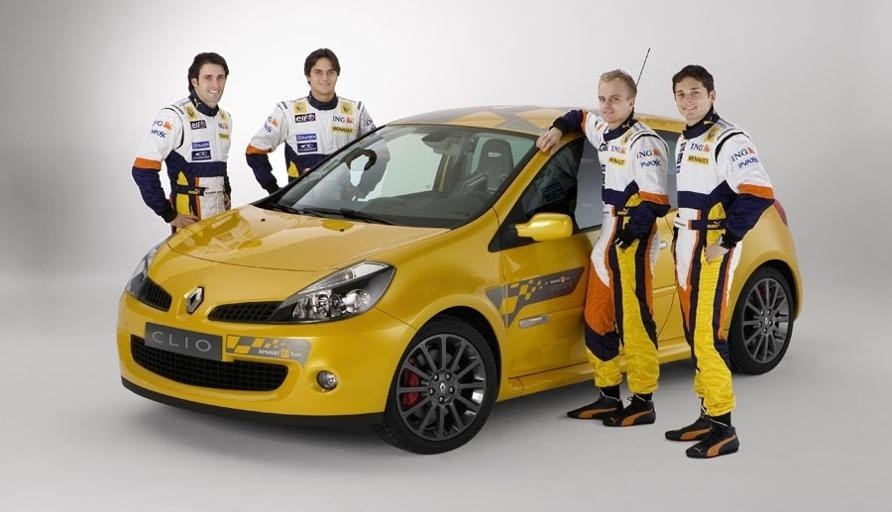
[115,48,803,455]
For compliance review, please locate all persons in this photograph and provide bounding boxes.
[246,49,391,206]
[536,70,671,426]
[132,53,233,235]
[665,65,775,457]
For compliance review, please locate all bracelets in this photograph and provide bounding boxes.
[716,234,736,249]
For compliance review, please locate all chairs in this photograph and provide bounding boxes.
[450,138,515,197]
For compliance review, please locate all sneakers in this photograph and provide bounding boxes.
[604,395,655,425]
[685,427,740,458]
[567,391,625,420]
[665,415,712,440]
